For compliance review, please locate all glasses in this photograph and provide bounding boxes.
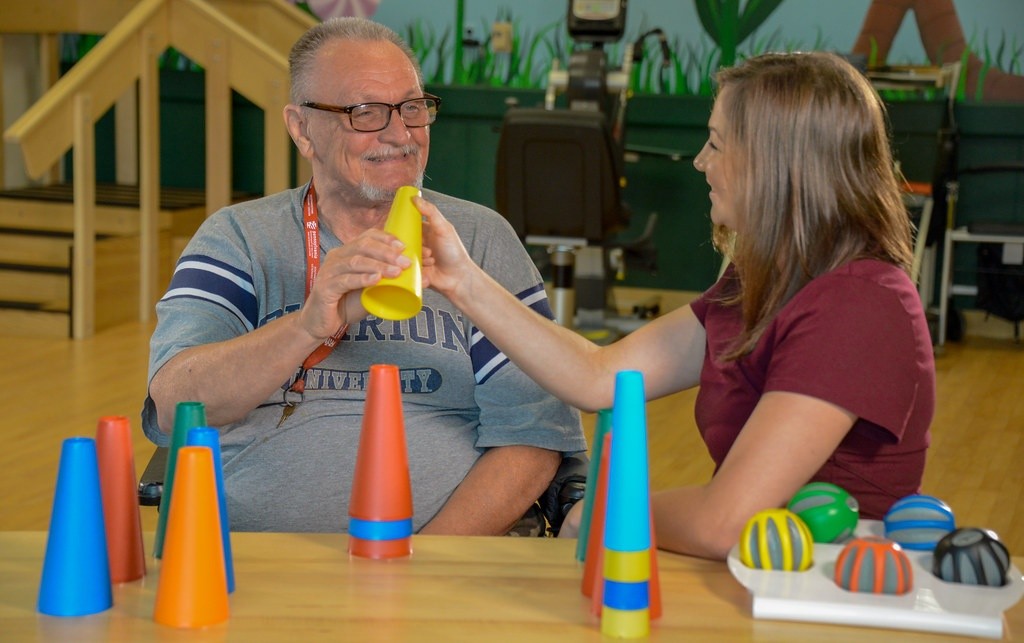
[296,89,441,135]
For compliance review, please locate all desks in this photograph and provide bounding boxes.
[3,526,1024,642]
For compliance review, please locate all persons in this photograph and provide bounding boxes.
[413,53,936,560]
[141,17,587,539]
